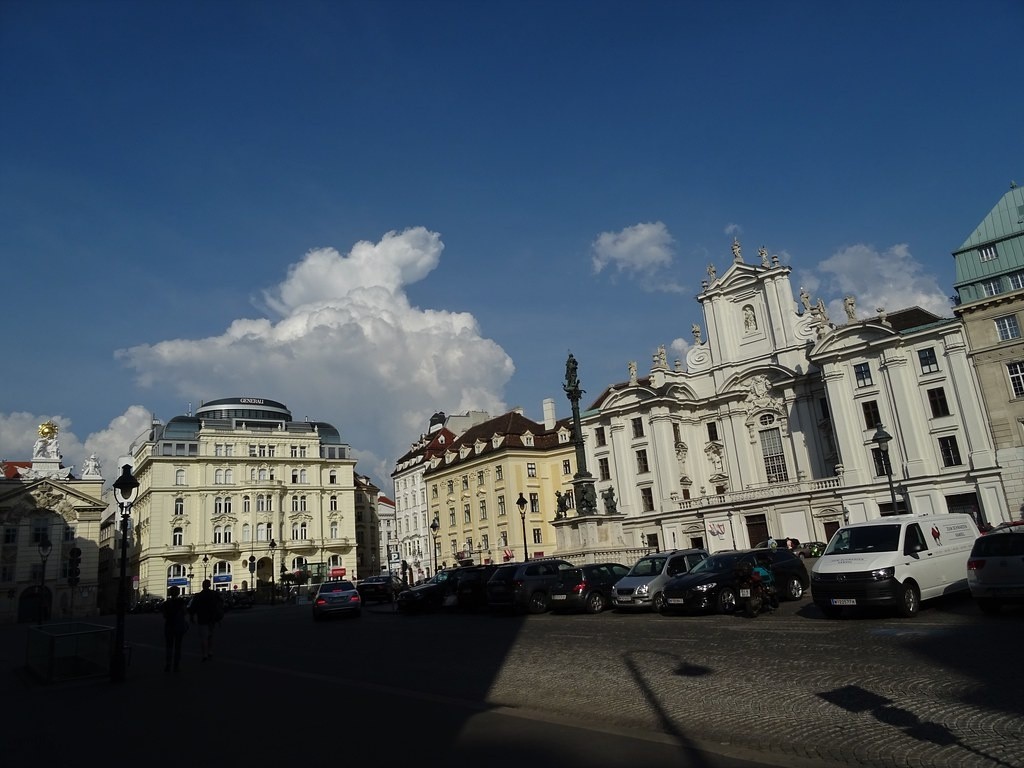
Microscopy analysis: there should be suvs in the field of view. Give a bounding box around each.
[660,547,810,616]
[487,560,576,614]
[396,565,490,614]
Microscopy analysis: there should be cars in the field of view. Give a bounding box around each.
[311,579,362,621]
[793,542,828,561]
[551,563,641,614]
[966,520,1024,615]
[356,575,408,605]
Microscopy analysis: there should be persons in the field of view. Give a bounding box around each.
[417,569,425,583]
[731,241,741,258]
[707,264,716,282]
[758,248,767,261]
[744,307,755,328]
[692,325,701,344]
[659,344,667,365]
[799,290,856,322]
[767,536,793,551]
[628,362,637,380]
[554,487,617,517]
[159,580,223,672]
[565,353,578,387]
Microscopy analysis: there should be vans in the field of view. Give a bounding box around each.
[610,549,711,613]
[754,539,802,554]
[811,512,983,618]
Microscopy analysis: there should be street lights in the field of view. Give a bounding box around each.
[202,554,209,580]
[430,519,440,583]
[268,539,277,603]
[37,533,53,629]
[106,464,141,684]
[640,533,645,547]
[872,423,899,515]
[726,510,737,550]
[515,493,529,562]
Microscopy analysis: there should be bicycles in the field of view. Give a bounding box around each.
[130,590,258,615]
